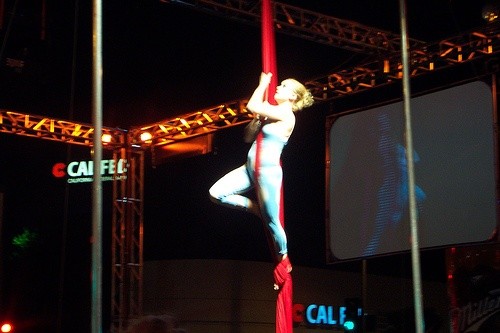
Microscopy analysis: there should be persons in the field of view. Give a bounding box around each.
[208,72,313,291]
